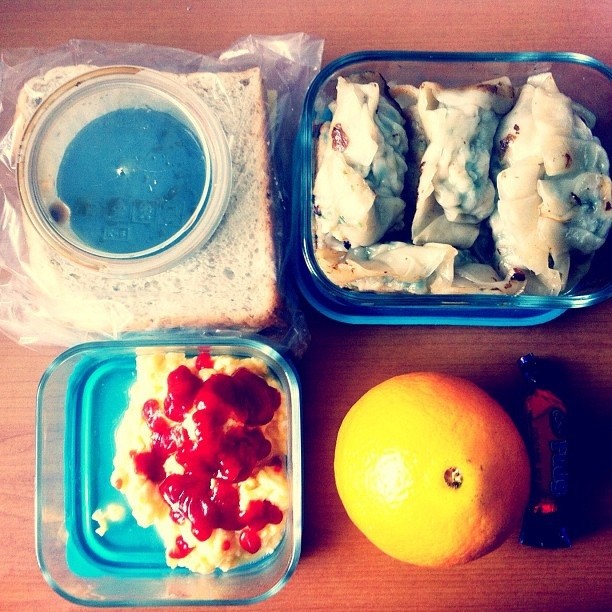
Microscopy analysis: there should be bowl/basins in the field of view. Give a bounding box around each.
[33,330,301,609]
[299,49,612,313]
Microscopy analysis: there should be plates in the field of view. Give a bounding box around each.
[298,273,581,328]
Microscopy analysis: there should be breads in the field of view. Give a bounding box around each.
[13,64,279,335]
